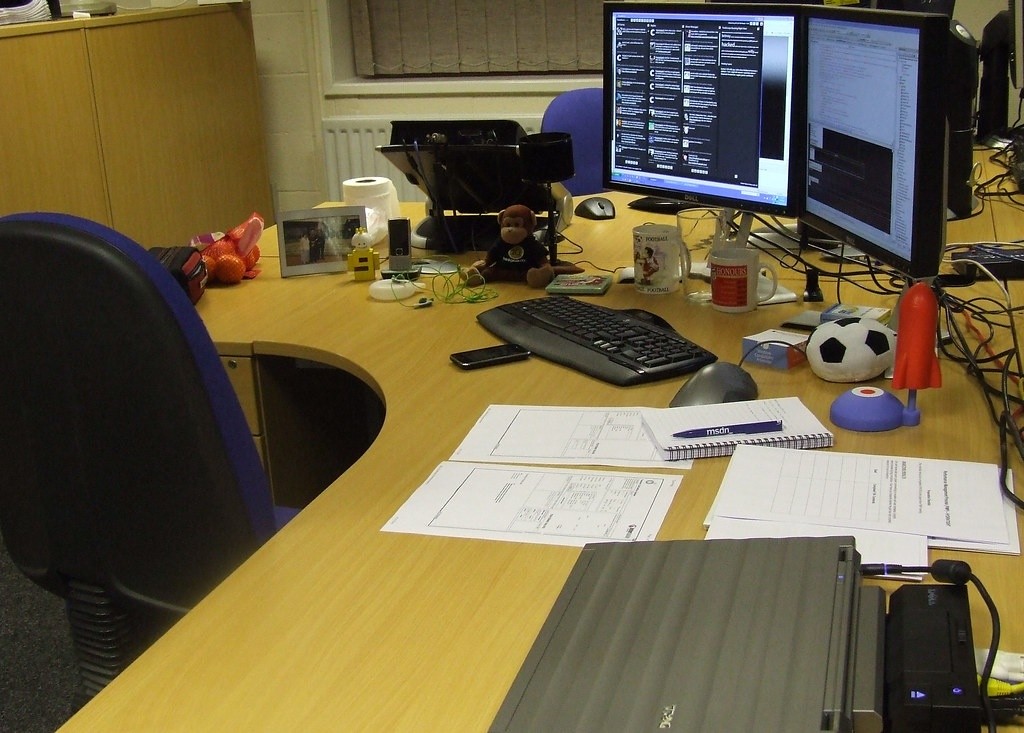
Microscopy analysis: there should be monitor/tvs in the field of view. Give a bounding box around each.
[602,0,801,219]
[799,4,949,277]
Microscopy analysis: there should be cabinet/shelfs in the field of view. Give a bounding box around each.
[0,0,275,252]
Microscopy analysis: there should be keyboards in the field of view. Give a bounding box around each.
[476,294,719,387]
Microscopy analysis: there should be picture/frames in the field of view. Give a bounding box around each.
[274,206,368,278]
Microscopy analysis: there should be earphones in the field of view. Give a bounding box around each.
[390,275,409,284]
[414,298,432,308]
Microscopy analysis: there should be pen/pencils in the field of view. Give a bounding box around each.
[670,420,783,438]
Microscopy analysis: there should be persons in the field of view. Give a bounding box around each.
[300,232,311,265]
[307,228,324,263]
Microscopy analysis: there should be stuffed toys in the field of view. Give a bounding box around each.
[458,204,585,289]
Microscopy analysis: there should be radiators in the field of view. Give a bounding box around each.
[322,115,544,203]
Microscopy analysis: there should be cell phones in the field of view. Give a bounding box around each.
[450,343,531,370]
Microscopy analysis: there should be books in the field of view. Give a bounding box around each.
[640,397,834,462]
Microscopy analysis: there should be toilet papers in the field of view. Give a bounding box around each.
[342,176,401,231]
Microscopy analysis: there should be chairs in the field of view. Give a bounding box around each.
[542,88,603,198]
[0,212,302,717]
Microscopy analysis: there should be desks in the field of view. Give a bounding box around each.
[53,141,1024,733]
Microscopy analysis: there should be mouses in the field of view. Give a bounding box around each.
[668,362,758,406]
[574,197,614,220]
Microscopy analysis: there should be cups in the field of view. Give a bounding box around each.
[632,225,691,294]
[709,247,777,313]
